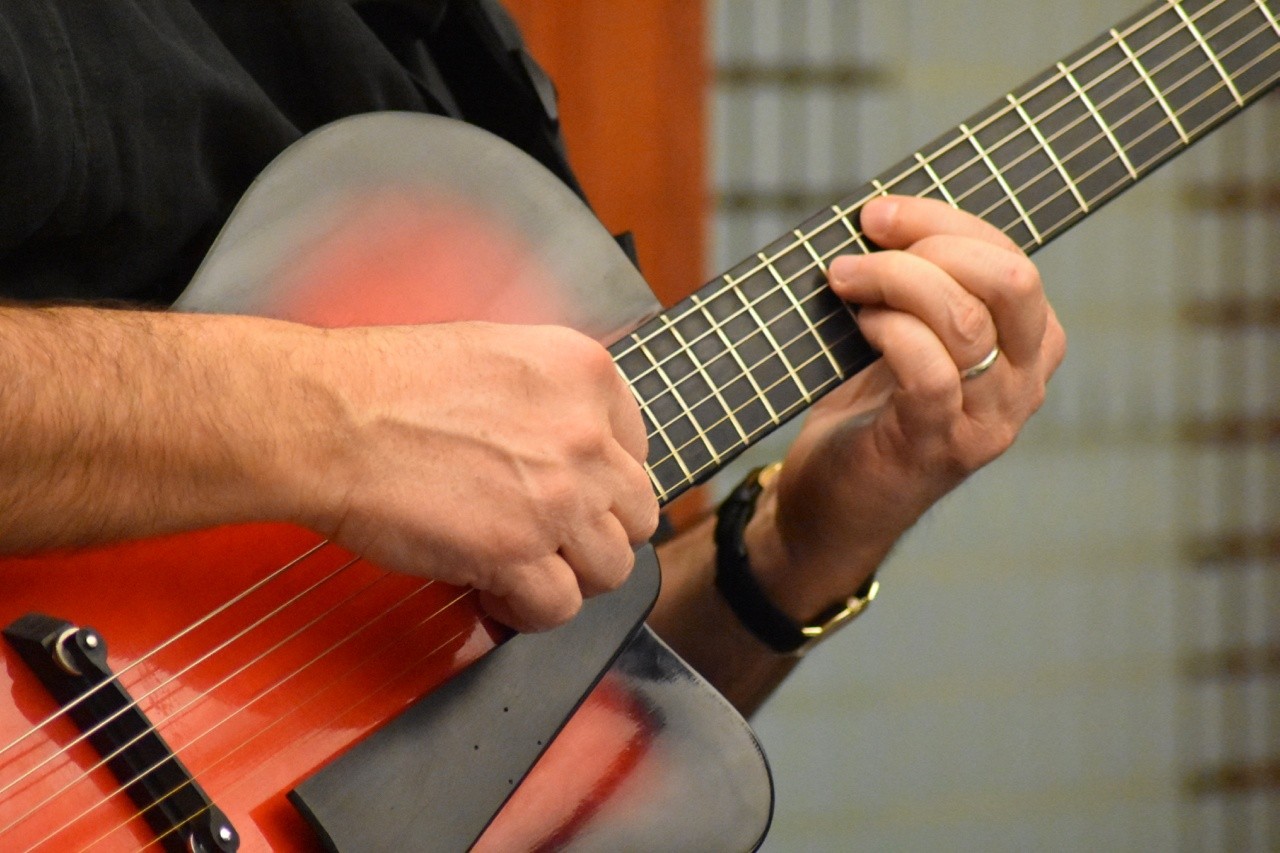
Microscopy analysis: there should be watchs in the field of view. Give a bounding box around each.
[707,452,885,663]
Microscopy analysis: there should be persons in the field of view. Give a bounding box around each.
[1,0,1071,722]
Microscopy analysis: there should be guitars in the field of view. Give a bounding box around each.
[1,1,1280,853]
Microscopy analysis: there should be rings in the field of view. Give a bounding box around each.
[960,344,1000,382]
